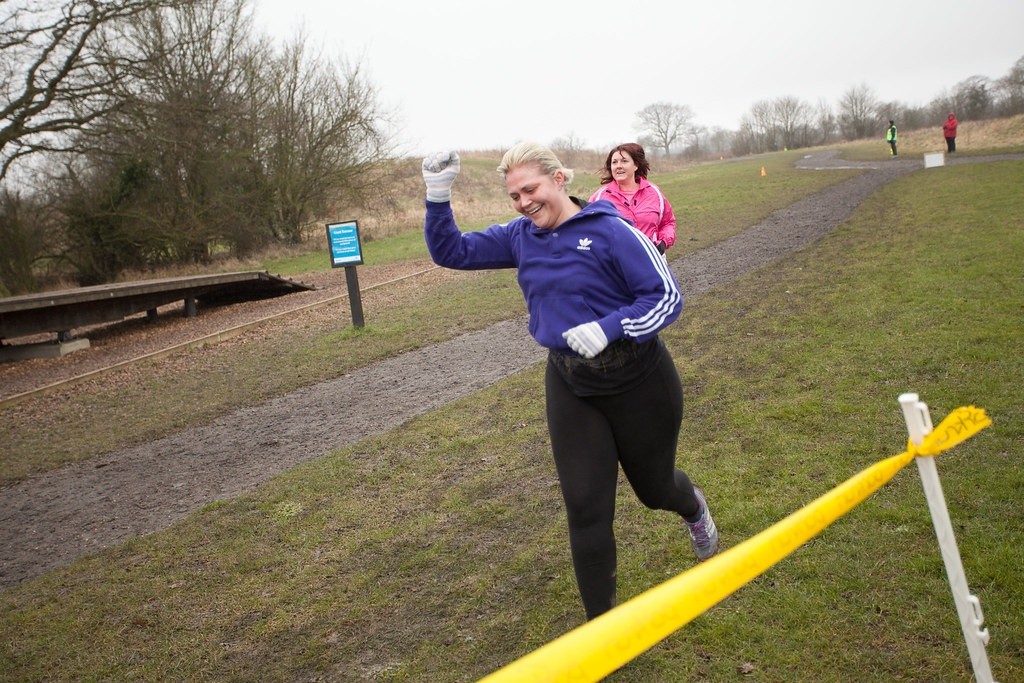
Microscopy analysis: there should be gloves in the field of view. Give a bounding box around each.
[561,321,608,360]
[422,148,461,203]
[652,240,665,256]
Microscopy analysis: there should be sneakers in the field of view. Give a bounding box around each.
[685,489,717,558]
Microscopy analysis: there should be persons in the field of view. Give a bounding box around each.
[942,111,958,154]
[421,141,717,624]
[588,143,677,266]
[887,120,898,158]
